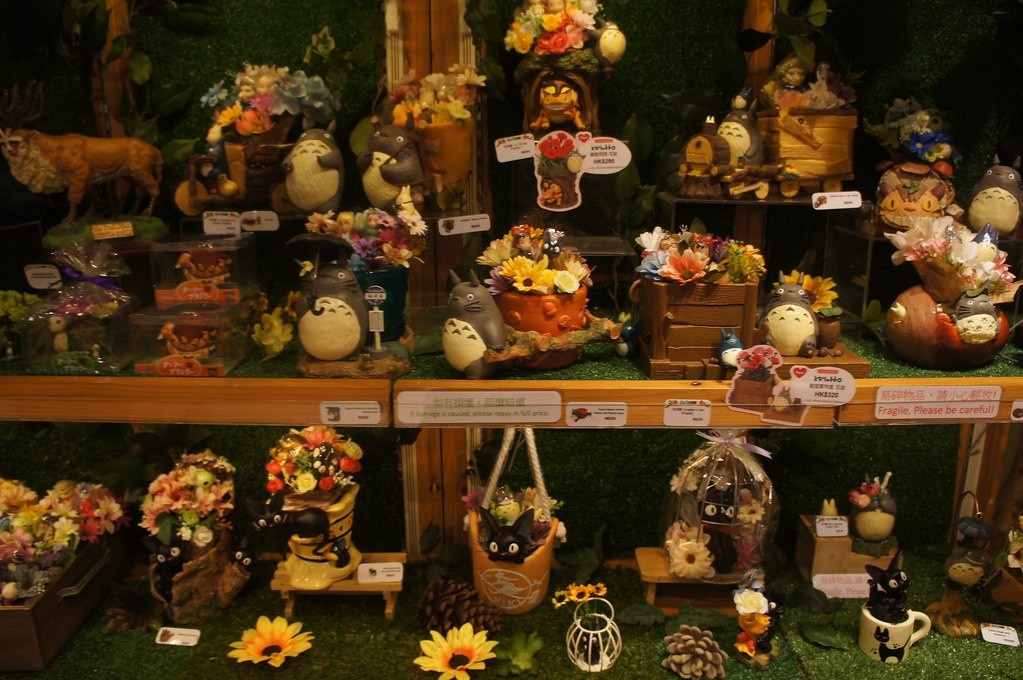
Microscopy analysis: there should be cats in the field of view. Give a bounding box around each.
[246,490,330,556]
[697,480,739,575]
[477,504,534,565]
[865,548,911,625]
[230,534,257,571]
[141,534,184,602]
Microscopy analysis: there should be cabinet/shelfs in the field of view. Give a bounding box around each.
[824,215,899,326]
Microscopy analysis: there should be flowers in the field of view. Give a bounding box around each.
[1,1,1022,679]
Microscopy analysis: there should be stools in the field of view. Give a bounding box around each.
[270,551,407,621]
[634,547,766,606]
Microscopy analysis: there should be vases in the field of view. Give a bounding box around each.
[567,599,625,672]
[344,264,409,342]
[856,507,897,541]
[814,305,846,350]
[628,277,759,379]
[0,533,125,671]
[225,114,342,205]
[420,117,475,187]
[274,482,364,590]
[886,285,1016,371]
[676,485,769,572]
[150,532,250,626]
[990,565,1022,604]
[855,217,880,239]
[494,269,590,370]
[473,517,560,614]
[758,109,858,179]
